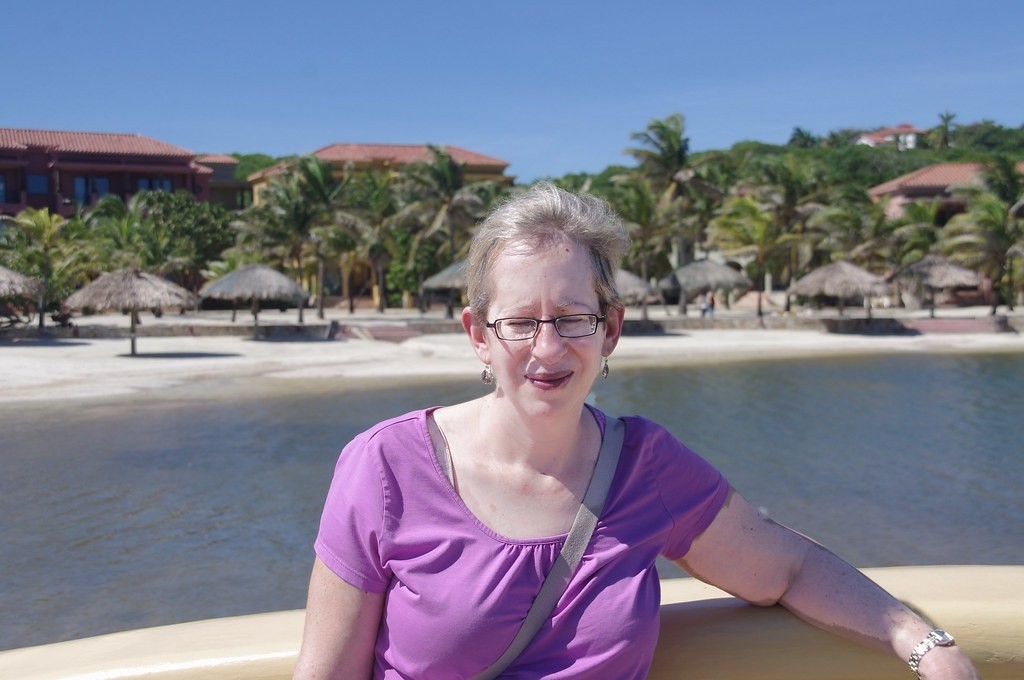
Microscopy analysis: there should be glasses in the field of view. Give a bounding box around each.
[483,314,607,341]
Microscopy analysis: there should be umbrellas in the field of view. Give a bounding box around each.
[0,265,45,297]
[658,258,751,319]
[198,265,309,321]
[617,268,657,297]
[65,267,202,353]
[885,254,981,318]
[790,259,893,319]
[424,261,469,288]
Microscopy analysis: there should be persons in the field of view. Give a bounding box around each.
[699,291,715,315]
[293,184,976,680]
[0,296,35,323]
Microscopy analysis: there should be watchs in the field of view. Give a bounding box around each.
[908,629,955,678]
